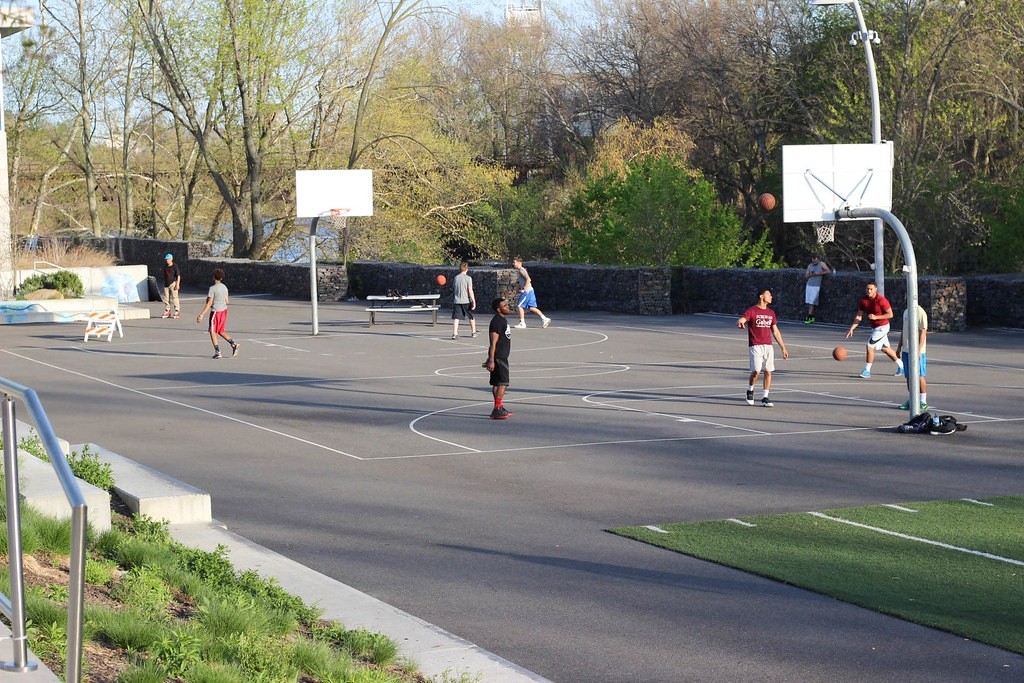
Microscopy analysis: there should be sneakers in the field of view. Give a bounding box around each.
[761,397,774,407]
[899,400,928,413]
[859,369,872,379]
[895,367,905,376]
[746,389,754,406]
[490,407,514,419]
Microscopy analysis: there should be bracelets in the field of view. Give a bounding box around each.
[199,314,203,318]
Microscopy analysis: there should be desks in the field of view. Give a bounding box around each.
[367,294,441,327]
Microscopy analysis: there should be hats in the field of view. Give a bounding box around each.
[165,254,173,259]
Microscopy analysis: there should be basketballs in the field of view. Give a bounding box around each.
[206,297,212,308]
[832,346,848,361]
[435,275,447,286]
[758,192,776,211]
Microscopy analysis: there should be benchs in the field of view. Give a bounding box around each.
[365,305,441,311]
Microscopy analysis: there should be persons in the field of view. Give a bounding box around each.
[805,257,831,324]
[845,281,904,378]
[897,291,929,413]
[512,256,551,329]
[482,298,514,420]
[162,253,180,319]
[197,269,239,358]
[450,262,480,340]
[737,289,788,407]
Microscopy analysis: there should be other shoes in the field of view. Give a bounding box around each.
[452,334,460,340]
[542,318,552,328]
[231,342,240,356]
[802,316,816,324]
[162,311,172,319]
[212,351,223,359]
[472,332,480,338]
[514,322,527,329]
[173,312,180,318]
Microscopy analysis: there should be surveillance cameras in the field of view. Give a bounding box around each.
[873,38,881,45]
[850,39,857,47]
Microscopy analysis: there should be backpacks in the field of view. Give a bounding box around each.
[898,412,931,432]
